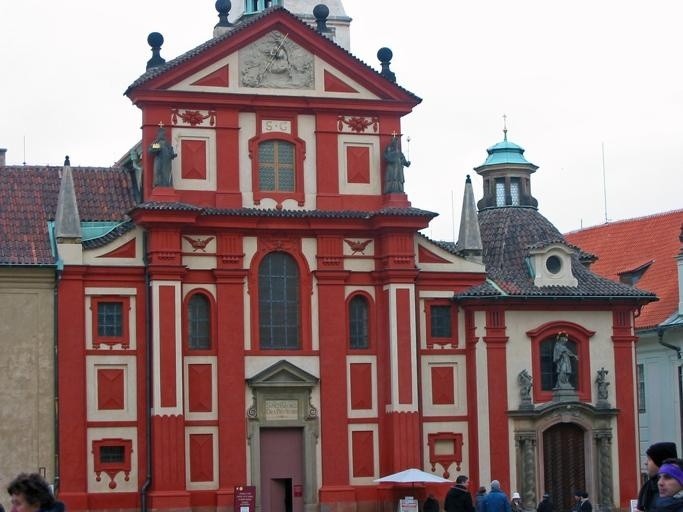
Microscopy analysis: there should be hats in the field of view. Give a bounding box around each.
[645,442,676,468]
[511,493,519,499]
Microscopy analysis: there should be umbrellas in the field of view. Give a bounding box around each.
[371,465,455,504]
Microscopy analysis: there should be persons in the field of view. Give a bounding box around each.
[381,134,412,196]
[146,125,178,190]
[636,441,683,511]
[6,472,67,510]
[647,458,682,511]
[422,473,594,511]
[551,328,582,401]
